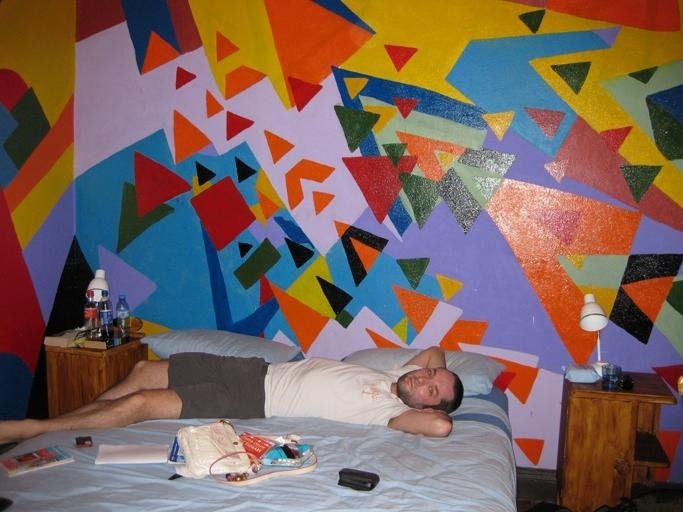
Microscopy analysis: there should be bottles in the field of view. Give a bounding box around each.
[83,289,102,338]
[116,294,130,343]
[96,290,114,347]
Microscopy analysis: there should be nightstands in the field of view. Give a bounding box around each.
[556,373,677,511]
[45,331,148,419]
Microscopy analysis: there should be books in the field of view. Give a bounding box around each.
[78,329,128,349]
[43,327,92,348]
[0,444,74,478]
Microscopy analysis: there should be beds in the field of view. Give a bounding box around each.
[1,386,516,512]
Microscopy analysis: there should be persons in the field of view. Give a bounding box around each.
[0,344,464,445]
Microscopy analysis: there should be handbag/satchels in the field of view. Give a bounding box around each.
[176,422,250,477]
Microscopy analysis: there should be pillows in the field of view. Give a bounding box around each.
[342,349,506,394]
[140,330,301,363]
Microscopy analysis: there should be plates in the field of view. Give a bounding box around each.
[126,316,142,333]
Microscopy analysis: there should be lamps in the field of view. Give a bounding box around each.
[85,269,110,302]
[580,293,608,377]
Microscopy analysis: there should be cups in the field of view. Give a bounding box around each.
[601,364,622,390]
